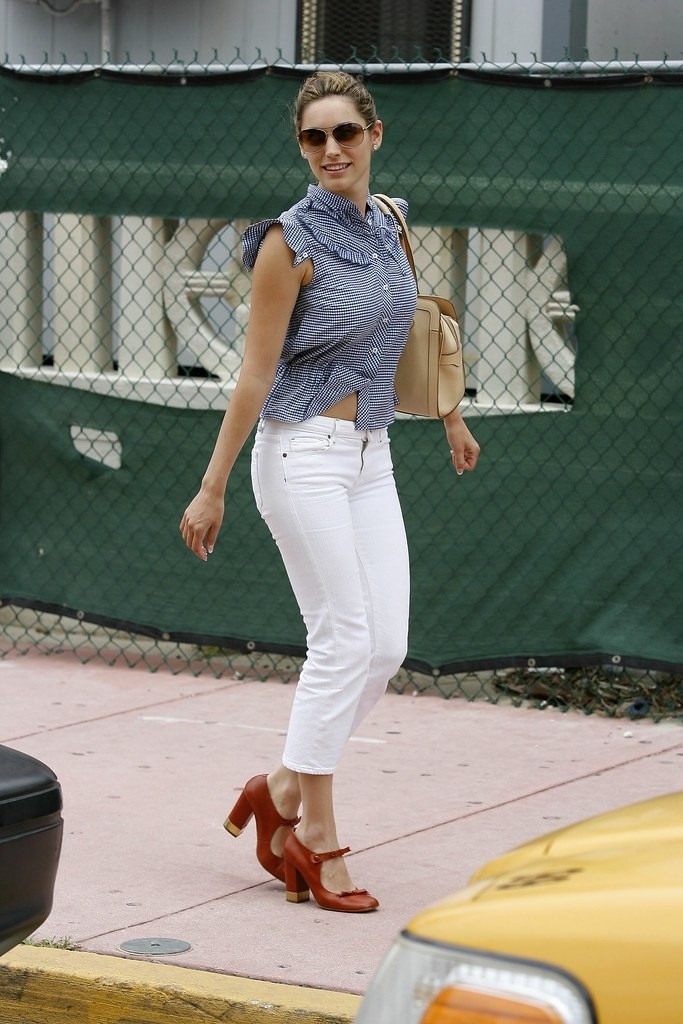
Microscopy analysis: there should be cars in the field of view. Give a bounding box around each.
[356,790,683,1022]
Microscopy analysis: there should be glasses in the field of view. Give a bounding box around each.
[297,122,374,154]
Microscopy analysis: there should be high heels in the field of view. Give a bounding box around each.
[223,772,302,884]
[283,829,380,914]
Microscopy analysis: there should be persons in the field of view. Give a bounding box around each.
[177,70,482,913]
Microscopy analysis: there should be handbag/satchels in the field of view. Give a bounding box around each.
[394,295,467,418]
